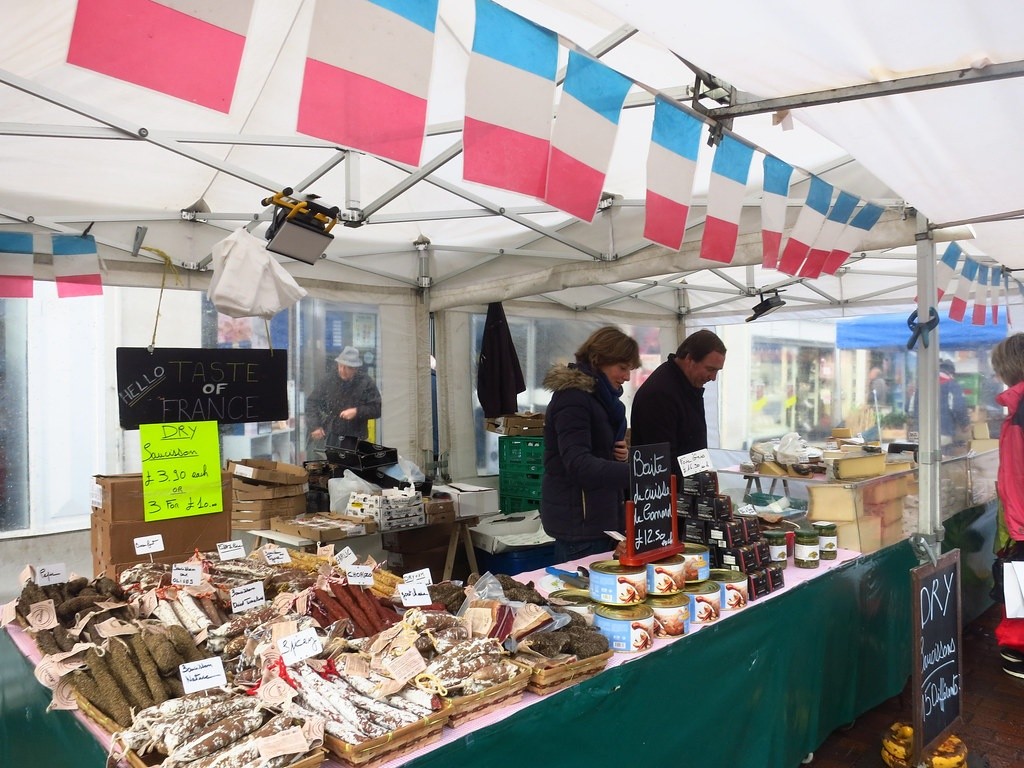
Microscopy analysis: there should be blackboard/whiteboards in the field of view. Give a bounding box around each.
[619,440,686,568]
[908,547,966,765]
[114,346,292,433]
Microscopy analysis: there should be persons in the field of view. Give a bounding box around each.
[304,345,383,441]
[914,359,972,448]
[538,324,634,562]
[868,365,888,406]
[631,326,727,492]
[990,330,1024,680]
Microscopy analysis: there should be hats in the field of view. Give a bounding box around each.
[333,346,363,368]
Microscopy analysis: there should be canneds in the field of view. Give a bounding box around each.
[762,520,837,570]
[548,542,749,653]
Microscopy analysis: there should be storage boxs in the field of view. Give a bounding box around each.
[91,409,557,586]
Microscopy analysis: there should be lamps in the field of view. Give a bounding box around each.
[745,296,787,322]
[265,207,335,266]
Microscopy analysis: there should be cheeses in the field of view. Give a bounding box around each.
[739,427,919,552]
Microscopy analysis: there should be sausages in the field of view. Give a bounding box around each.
[14,542,608,768]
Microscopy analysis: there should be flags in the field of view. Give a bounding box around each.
[972,262,989,326]
[538,49,635,226]
[460,1,561,201]
[913,240,964,305]
[1003,273,1014,326]
[65,0,255,113]
[822,200,886,275]
[991,265,1002,324]
[50,223,105,299]
[295,3,440,168]
[946,254,979,324]
[642,92,707,253]
[761,153,794,270]
[0,230,33,300]
[780,175,834,278]
[699,132,758,266]
[800,189,863,280]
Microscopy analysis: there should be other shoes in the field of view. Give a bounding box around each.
[999,647,1024,679]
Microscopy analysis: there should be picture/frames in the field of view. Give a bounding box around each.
[910,548,965,767]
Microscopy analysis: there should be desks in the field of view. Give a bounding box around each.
[0,448,1001,768]
[253,516,482,585]
